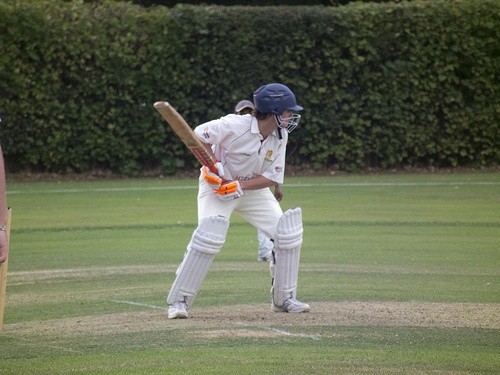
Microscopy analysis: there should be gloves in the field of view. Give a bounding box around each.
[200,161,224,190]
[217,179,243,201]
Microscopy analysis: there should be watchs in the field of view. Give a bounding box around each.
[0,223,7,231]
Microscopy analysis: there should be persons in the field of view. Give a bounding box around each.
[0,147,13,336]
[235,99,284,261]
[166,84,311,320]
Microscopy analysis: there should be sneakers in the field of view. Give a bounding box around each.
[167,301,188,318]
[271,298,310,313]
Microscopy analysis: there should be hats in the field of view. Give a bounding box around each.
[235,99,255,112]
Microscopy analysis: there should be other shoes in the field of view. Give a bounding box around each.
[258,251,275,261]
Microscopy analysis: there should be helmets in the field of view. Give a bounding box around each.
[253,83,303,115]
